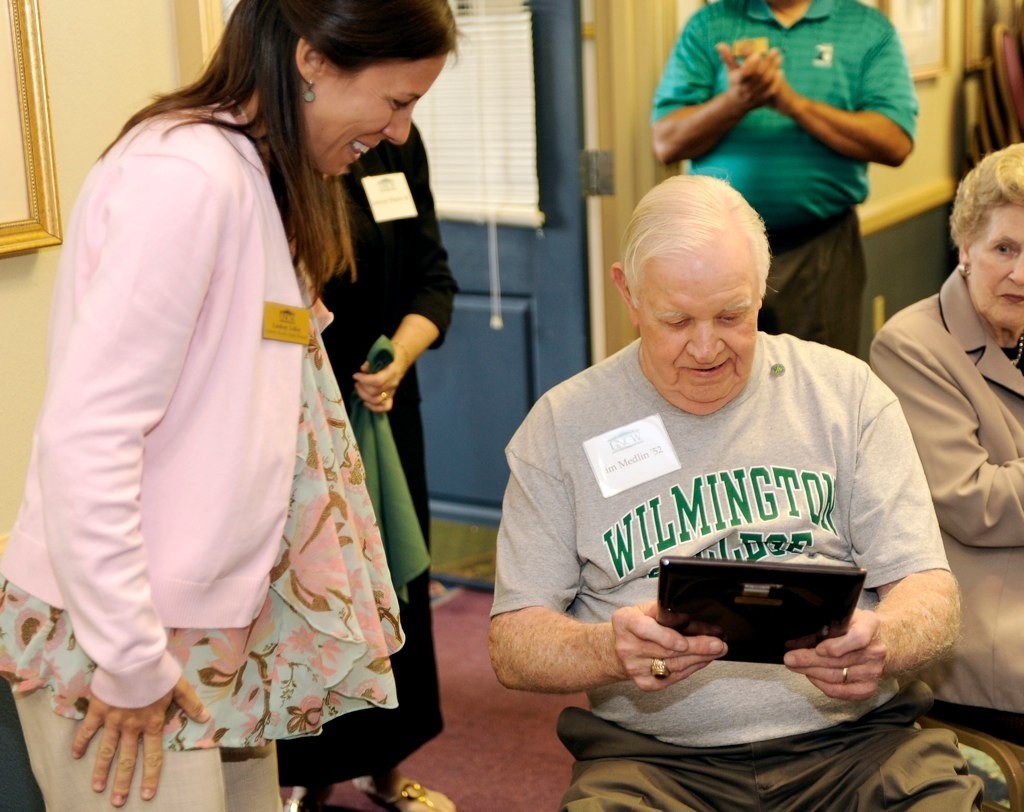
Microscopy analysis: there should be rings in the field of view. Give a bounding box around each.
[651,657,670,680]
[382,392,387,400]
[842,668,848,685]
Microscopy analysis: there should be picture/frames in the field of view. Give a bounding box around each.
[0,0,65,261]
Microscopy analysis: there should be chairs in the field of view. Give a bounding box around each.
[964,19,1024,163]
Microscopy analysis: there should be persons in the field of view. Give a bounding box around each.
[651,0,917,359]
[487,174,985,812]
[279,116,458,812]
[0,0,459,812]
[869,143,1024,713]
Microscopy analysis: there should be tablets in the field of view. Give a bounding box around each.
[655,557,868,664]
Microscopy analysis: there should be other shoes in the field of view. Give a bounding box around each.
[283,783,332,812]
[353,774,457,812]
